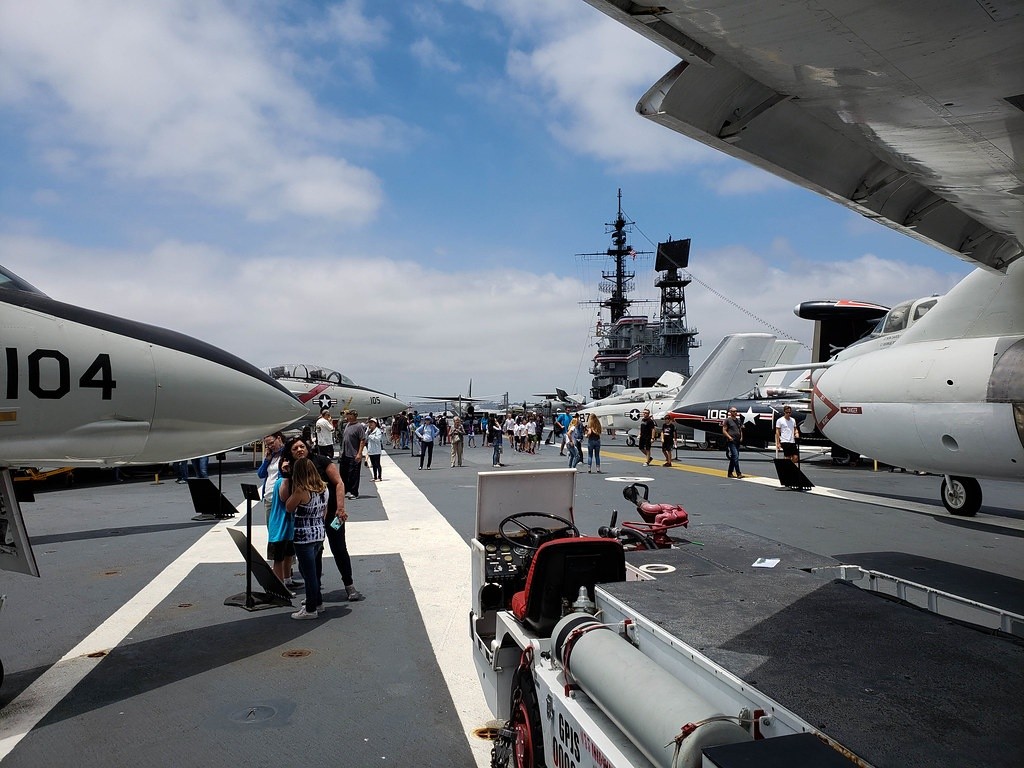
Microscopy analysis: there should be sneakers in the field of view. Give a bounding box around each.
[291,603,326,619]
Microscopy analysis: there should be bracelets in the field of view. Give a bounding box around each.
[651,438,654,439]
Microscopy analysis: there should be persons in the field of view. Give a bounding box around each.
[723,407,745,478]
[316,409,334,459]
[637,409,656,466]
[660,415,676,467]
[776,406,800,462]
[553,408,602,473]
[385,411,464,470]
[465,403,545,468]
[173,456,209,483]
[338,410,382,498]
[607,429,612,435]
[257,432,361,620]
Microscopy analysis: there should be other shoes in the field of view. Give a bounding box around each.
[344,584,360,600]
[560,452,566,456]
[643,456,654,467]
[369,479,382,482]
[663,463,672,467]
[178,480,188,485]
[728,474,745,479]
[510,444,535,455]
[468,445,477,448]
[286,580,306,597]
[418,467,431,470]
[578,461,583,465]
[493,462,504,468]
[344,491,359,500]
[451,464,461,468]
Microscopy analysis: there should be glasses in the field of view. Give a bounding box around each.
[784,410,792,414]
[665,417,671,420]
[265,436,279,447]
[286,434,303,443]
[730,410,738,413]
[642,411,650,414]
[566,409,572,412]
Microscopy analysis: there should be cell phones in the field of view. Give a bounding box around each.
[330,516,345,530]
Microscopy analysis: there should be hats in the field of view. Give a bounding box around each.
[347,410,358,417]
[368,418,377,423]
[425,416,432,422]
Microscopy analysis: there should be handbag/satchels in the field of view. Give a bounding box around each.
[451,430,460,443]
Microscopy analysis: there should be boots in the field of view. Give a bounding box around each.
[587,464,602,473]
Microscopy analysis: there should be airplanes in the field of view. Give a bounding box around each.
[527,294,942,466]
[582,0,1023,517]
[260,364,411,435]
[0,265,309,578]
